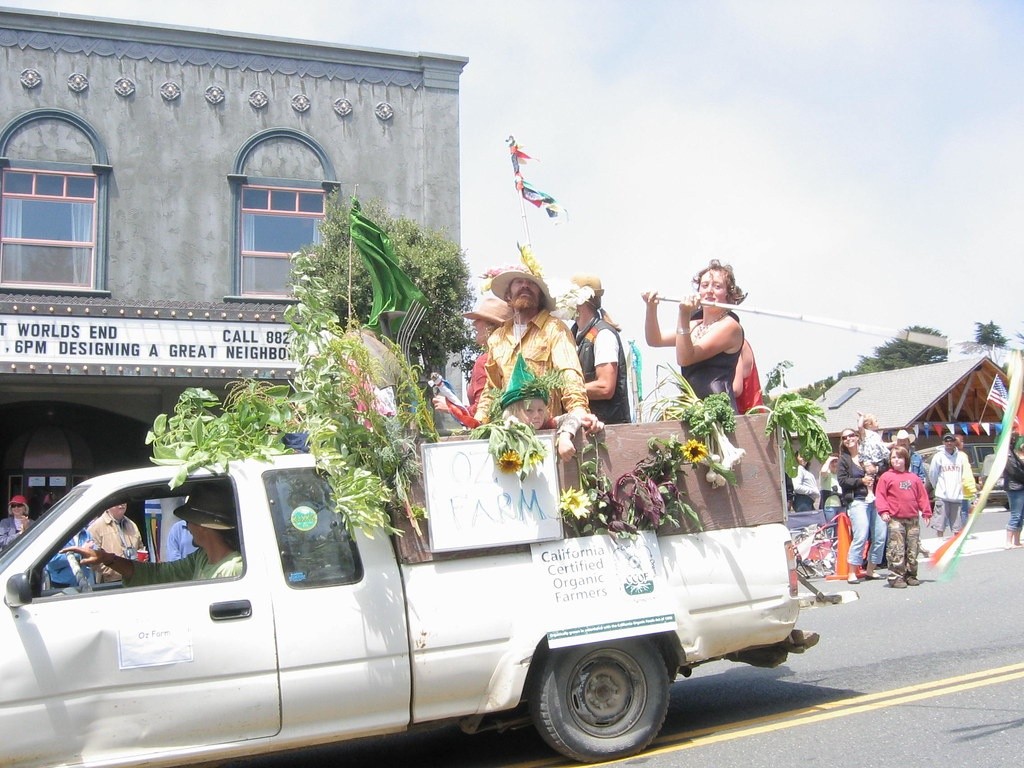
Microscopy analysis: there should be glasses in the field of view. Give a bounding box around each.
[11,504,24,508]
[943,438,954,443]
[841,433,856,441]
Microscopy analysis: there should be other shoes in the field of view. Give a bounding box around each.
[907,576,920,586]
[847,576,860,584]
[1005,542,1024,549]
[889,579,908,587]
[865,495,877,504]
[865,571,886,580]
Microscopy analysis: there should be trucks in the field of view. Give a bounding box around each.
[0,450,860,768]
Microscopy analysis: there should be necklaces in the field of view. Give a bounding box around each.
[692,311,727,340]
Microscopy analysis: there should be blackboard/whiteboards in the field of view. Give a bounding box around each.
[421,435,564,553]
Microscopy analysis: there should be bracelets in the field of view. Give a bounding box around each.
[676,326,691,334]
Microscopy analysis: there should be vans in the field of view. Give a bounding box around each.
[913,443,1011,511]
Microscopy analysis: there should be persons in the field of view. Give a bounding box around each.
[44,527,95,590]
[165,521,200,563]
[431,263,766,461]
[1003,433,1024,550]
[340,329,399,434]
[0,494,35,553]
[87,502,150,583]
[784,413,976,589]
[59,484,243,588]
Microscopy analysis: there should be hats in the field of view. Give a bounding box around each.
[490,266,557,311]
[8,495,27,506]
[890,429,916,445]
[499,353,549,409]
[173,481,238,530]
[462,294,507,326]
[572,278,604,297]
[943,433,955,440]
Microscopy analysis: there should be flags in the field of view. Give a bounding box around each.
[989,376,1009,413]
